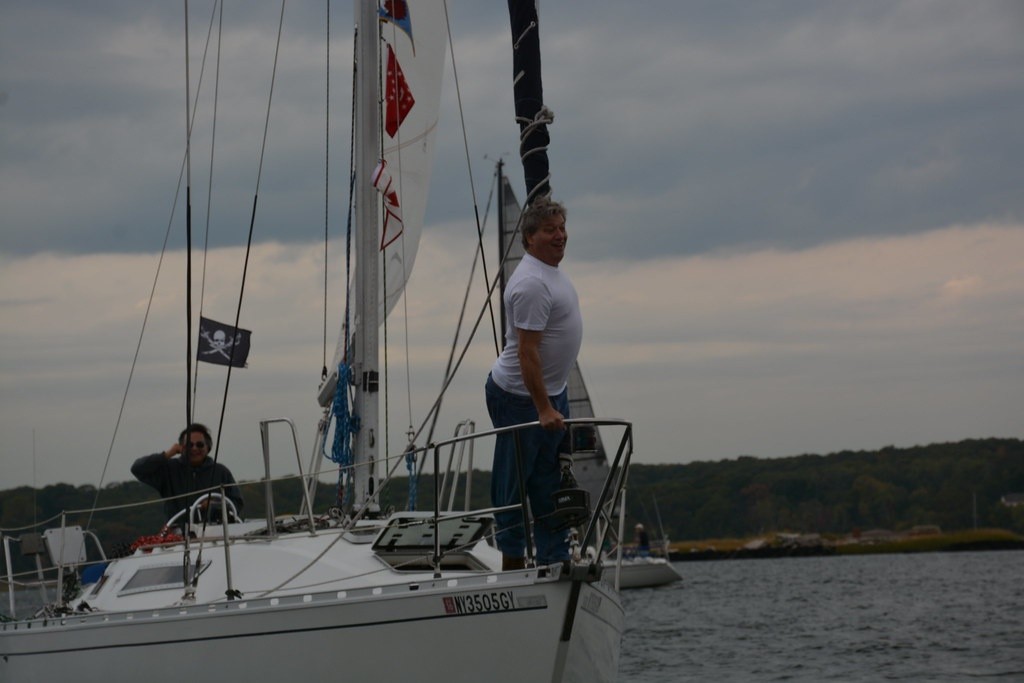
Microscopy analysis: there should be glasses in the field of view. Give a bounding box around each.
[190,441,208,448]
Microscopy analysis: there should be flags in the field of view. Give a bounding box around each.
[198,316,252,371]
[369,0,415,250]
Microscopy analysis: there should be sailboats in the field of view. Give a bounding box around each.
[402,158,682,589]
[0,11,635,682]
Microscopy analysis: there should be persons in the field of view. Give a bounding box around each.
[485,196,585,570]
[634,522,650,558]
[130,423,244,526]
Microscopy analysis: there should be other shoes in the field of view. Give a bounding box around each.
[536,562,596,578]
[502,554,526,571]
